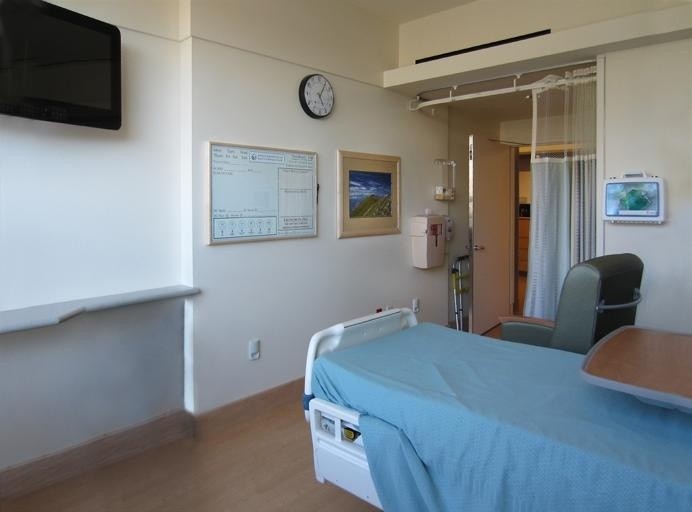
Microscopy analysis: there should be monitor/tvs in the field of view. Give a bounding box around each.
[0,0,122,131]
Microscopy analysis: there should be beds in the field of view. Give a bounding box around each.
[301,305,692,512]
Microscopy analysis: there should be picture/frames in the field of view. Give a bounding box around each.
[335,149,403,240]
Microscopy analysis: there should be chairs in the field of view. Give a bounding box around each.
[496,252,647,357]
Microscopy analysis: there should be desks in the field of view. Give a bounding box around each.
[578,322,691,412]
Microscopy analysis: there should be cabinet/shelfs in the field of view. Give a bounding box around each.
[516,217,530,272]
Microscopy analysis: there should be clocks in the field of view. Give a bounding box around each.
[297,71,337,120]
